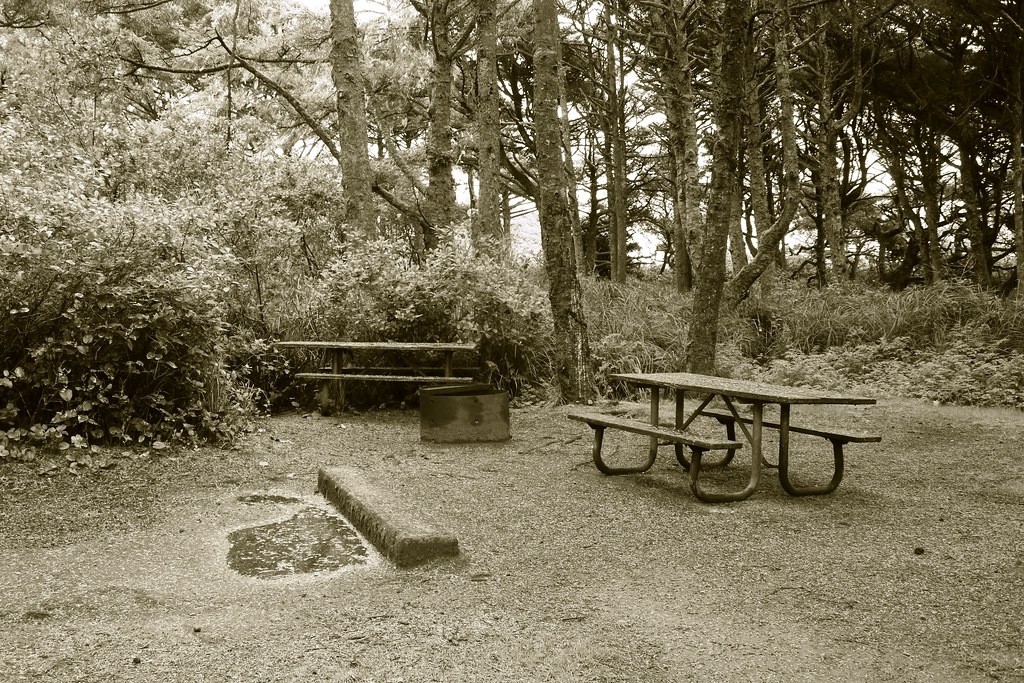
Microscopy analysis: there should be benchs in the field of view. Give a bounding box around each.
[294,373,473,418]
[566,413,743,504]
[319,365,481,406]
[696,408,881,494]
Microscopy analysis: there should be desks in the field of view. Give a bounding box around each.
[608,374,878,501]
[278,341,479,410]
